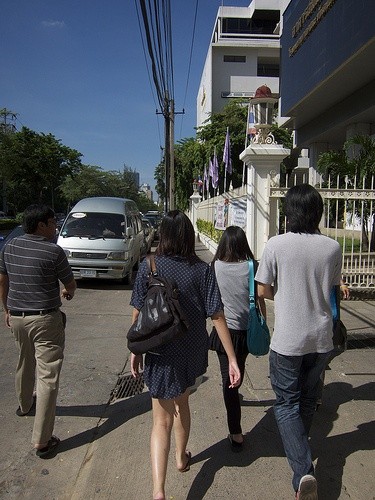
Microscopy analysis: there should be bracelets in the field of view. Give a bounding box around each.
[340,285,347,289]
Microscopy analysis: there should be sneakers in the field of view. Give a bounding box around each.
[295,474,319,500]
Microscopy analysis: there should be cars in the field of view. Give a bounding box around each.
[139,210,162,256]
[0,225,25,250]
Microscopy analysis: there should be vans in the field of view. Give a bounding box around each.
[52,197,147,284]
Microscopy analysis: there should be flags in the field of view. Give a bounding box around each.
[199,108,254,190]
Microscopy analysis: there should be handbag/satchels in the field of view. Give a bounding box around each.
[127,255,191,355]
[332,317,347,353]
[246,259,270,356]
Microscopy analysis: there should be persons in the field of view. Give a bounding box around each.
[252,184,351,500]
[129,210,241,500]
[0,205,77,455]
[209,225,269,449]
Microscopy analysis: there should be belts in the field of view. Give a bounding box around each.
[7,307,59,316]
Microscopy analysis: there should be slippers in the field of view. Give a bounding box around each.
[177,450,191,471]
[227,432,245,453]
[36,435,61,456]
[16,390,36,416]
